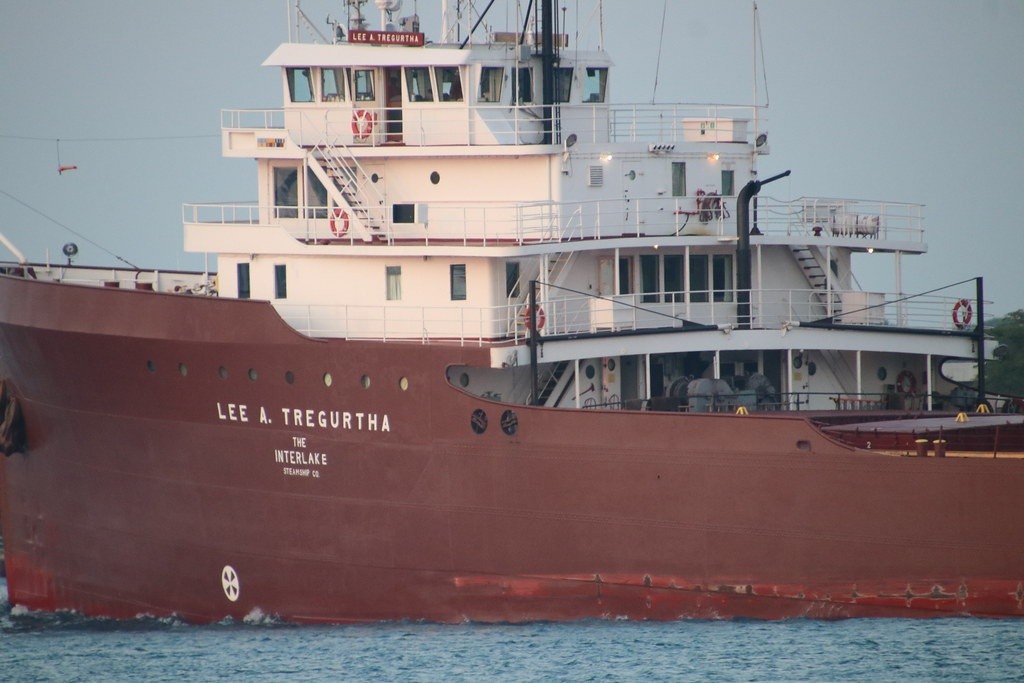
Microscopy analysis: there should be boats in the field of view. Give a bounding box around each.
[0,0,1023,625]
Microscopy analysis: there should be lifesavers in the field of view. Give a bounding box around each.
[952,298,973,329]
[351,109,373,140]
[524,304,545,333]
[896,369,918,400]
[328,208,350,238]
